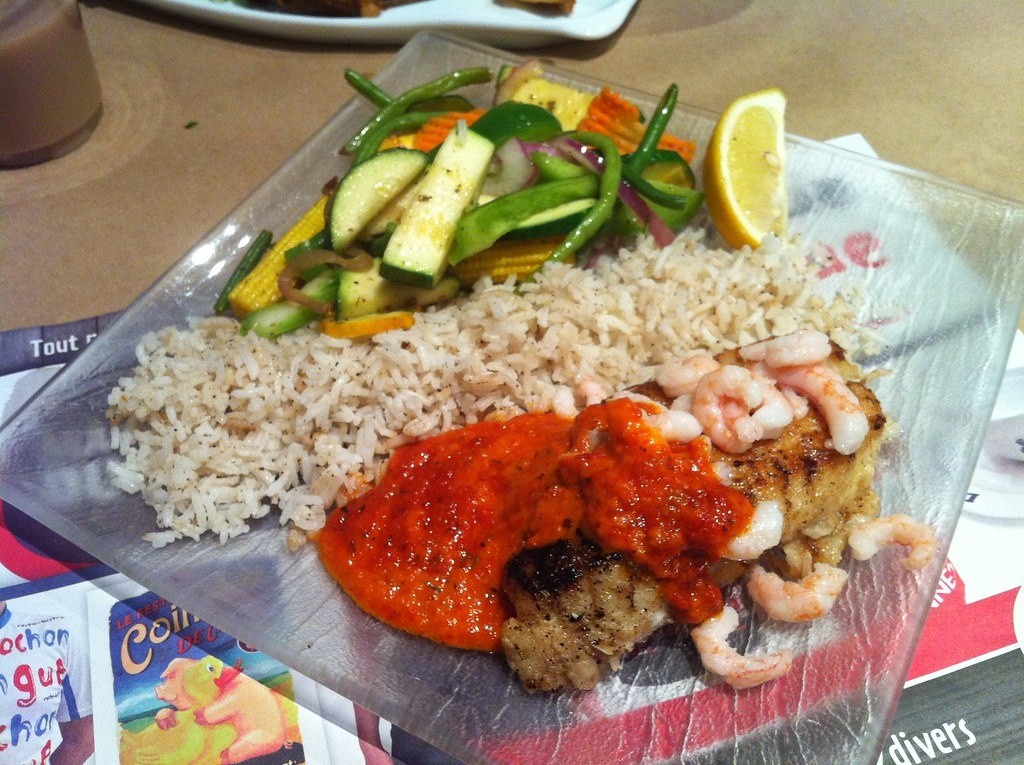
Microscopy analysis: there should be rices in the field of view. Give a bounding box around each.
[110,226,888,549]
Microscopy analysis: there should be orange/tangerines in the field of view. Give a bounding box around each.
[706,88,786,249]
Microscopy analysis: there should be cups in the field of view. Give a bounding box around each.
[0,1,104,173]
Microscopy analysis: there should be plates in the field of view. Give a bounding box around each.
[139,0,640,49]
[0,25,1024,764]
